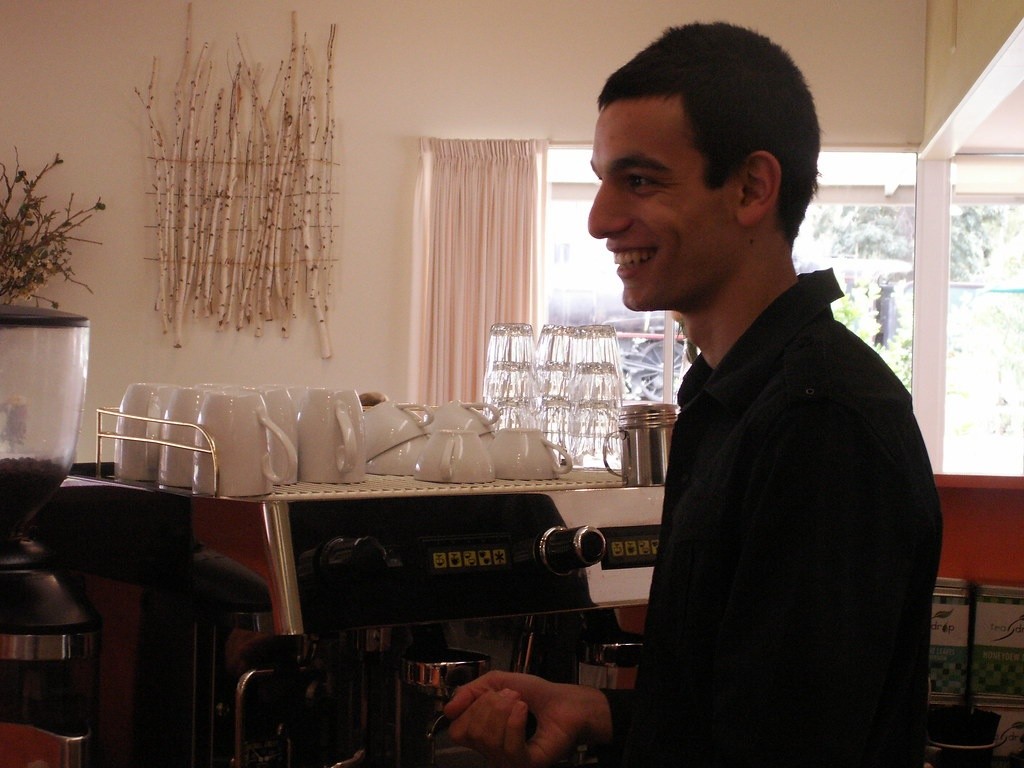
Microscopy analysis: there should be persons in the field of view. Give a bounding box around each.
[441,22,943,768]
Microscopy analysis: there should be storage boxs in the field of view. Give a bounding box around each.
[970,577,1024,697]
[929,577,974,696]
[972,695,1024,768]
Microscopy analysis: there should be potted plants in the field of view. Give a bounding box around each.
[0,142,105,475]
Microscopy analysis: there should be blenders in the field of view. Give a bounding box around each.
[0,303,100,738]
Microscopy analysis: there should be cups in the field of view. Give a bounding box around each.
[604,403,677,489]
[115,323,677,497]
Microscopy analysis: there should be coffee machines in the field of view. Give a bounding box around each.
[105,465,663,768]
